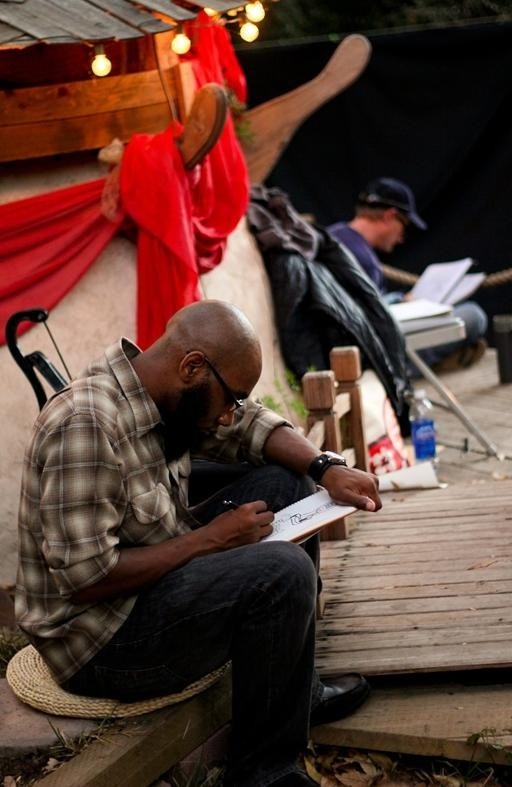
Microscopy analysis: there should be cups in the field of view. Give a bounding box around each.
[489,313,511,383]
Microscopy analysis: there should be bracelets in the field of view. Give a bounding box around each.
[309,448,348,484]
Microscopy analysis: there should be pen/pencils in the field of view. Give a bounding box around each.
[222,500,239,509]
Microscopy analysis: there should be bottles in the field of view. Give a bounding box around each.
[408,387,440,476]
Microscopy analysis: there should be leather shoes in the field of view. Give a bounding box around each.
[310,673,372,724]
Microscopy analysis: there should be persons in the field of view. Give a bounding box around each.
[317,177,491,376]
[17,298,382,785]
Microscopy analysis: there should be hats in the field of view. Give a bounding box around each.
[360,176,427,231]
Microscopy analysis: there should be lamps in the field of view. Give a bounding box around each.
[91,0,266,78]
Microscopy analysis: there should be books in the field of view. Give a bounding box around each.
[388,257,487,332]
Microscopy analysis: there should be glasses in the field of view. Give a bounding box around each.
[200,357,244,413]
